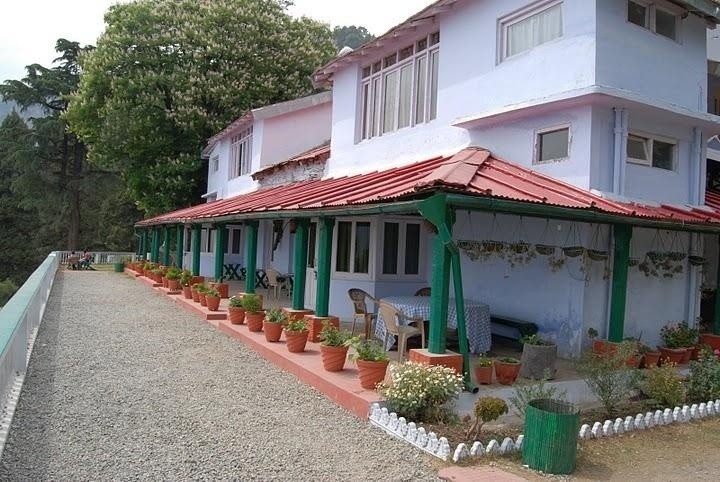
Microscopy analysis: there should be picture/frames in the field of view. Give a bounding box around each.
[373,293,492,353]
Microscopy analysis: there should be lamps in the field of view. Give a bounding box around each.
[376,302,426,361]
[67,253,97,271]
[347,289,380,339]
[413,286,432,336]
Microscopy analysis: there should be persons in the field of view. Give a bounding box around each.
[69,250,78,268]
[79,250,89,265]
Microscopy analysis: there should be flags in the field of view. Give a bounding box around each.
[474,366,494,386]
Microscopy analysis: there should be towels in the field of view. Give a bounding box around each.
[521,399,581,475]
[114,263,124,272]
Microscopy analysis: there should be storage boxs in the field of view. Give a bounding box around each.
[485,313,539,352]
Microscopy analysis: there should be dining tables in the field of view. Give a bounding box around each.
[515,330,559,380]
[494,353,528,385]
[583,323,719,369]
[121,258,385,392]
[456,237,712,283]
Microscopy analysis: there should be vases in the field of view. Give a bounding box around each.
[473,350,493,367]
